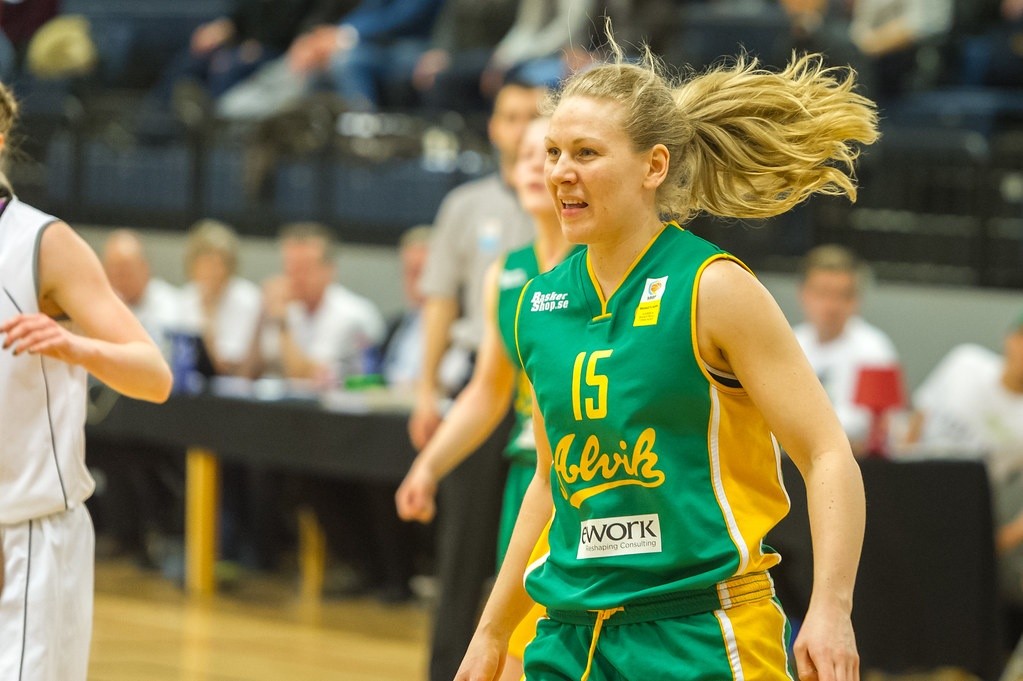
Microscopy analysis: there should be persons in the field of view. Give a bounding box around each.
[96,221,474,603]
[451,62,866,681]
[389,59,586,681]
[1,84,174,681]
[792,242,903,458]
[843,1,960,100]
[895,321,1023,595]
[132,0,602,113]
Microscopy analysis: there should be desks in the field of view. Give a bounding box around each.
[80,365,1023,609]
